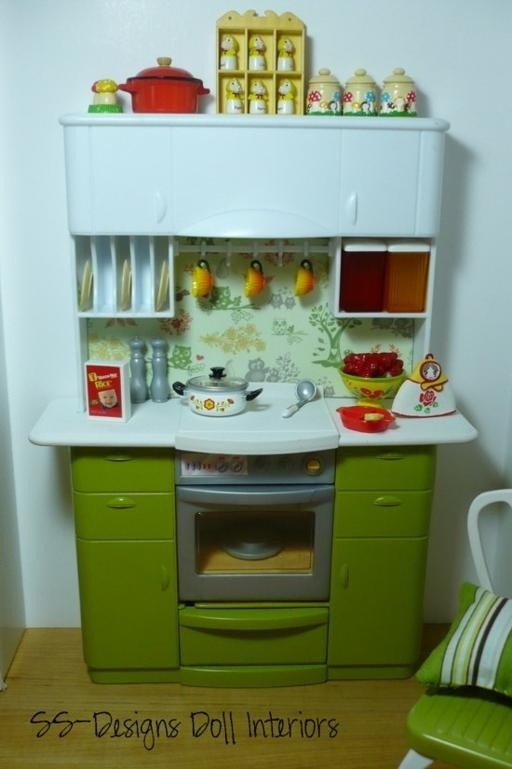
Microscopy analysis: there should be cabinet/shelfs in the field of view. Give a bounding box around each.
[26,113,480,690]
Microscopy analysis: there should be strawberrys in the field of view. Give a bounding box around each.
[344,352,404,377]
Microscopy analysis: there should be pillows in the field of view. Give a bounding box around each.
[414,580,511,698]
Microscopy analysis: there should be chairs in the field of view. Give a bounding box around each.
[397,487,512,769]
